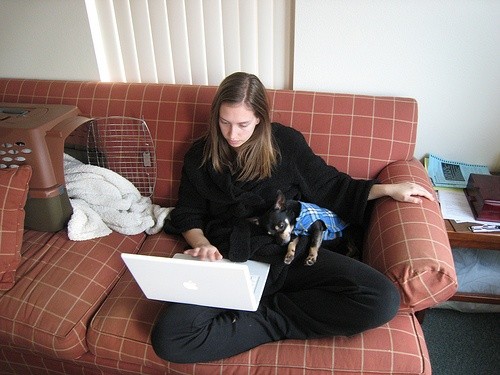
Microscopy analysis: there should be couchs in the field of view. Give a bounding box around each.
[0,77,459,375]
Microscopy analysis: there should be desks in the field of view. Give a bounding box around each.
[414,172,500,325]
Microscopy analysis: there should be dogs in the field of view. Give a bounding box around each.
[247,190,358,267]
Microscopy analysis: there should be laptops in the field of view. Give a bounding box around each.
[120,252,271,311]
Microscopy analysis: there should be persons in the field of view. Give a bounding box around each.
[151,72,435,363]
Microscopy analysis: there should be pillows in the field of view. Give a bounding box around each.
[0,165,32,290]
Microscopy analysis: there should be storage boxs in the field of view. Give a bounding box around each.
[464,173,500,223]
